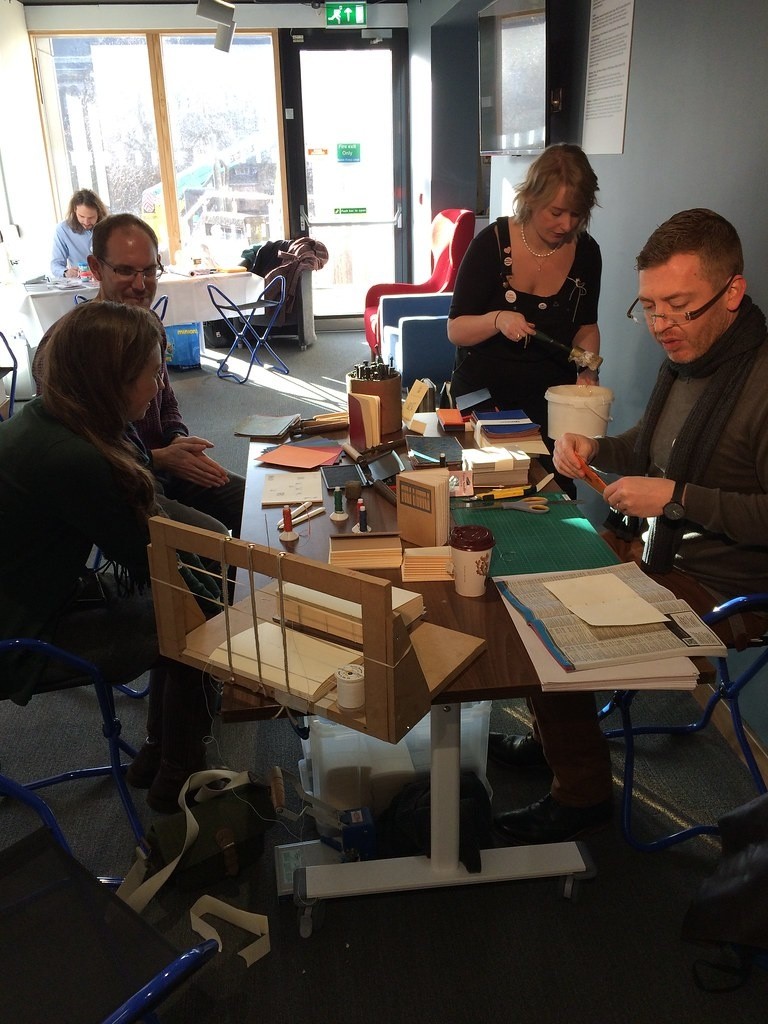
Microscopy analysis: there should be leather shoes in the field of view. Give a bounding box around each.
[487,731,558,772]
[489,790,617,846]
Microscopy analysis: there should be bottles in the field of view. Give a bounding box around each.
[78,262,90,282]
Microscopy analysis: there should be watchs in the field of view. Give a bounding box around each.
[663,480,686,522]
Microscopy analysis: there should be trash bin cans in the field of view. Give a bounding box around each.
[203,318,235,349]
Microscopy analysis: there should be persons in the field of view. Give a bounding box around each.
[50,189,107,279]
[0,302,234,812]
[446,142,602,508]
[31,214,245,612]
[486,208,768,845]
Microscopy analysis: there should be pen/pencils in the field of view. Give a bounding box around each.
[277,502,312,525]
[279,507,326,529]
[439,452,448,468]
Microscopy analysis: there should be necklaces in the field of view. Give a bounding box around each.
[530,254,544,271]
[521,219,565,257]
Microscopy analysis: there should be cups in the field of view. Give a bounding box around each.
[450,524,496,597]
[345,370,402,435]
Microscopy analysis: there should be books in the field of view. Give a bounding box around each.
[209,584,424,701]
[396,467,448,546]
[328,531,403,570]
[493,560,729,693]
[261,471,324,504]
[408,408,550,487]
[234,412,302,438]
[418,378,437,410]
[401,546,454,581]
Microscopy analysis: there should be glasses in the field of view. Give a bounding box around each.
[626,274,739,326]
[94,255,158,279]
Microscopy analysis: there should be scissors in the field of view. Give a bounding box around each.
[469,497,550,514]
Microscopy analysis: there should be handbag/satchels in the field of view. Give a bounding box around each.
[161,321,203,373]
[202,318,234,348]
[139,779,279,898]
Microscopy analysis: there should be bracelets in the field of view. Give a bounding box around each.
[494,310,502,331]
[63,270,67,278]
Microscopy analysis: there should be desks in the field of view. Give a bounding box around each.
[222,413,716,938]
[22,264,265,338]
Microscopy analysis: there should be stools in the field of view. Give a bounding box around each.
[207,275,290,384]
[0,332,18,422]
[75,294,168,321]
[599,593,768,853]
[1,774,219,1024]
[0,639,152,915]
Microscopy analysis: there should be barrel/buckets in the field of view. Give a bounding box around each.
[544,384,614,441]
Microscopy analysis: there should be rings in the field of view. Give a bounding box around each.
[517,334,520,339]
[616,503,623,512]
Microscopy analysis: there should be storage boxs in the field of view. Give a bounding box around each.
[298,699,493,836]
[0,395,10,420]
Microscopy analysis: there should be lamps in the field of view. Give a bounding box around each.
[195,0,237,52]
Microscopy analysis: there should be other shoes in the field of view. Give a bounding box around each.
[125,734,235,815]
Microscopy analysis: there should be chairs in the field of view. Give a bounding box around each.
[364,209,476,361]
[379,293,457,394]
